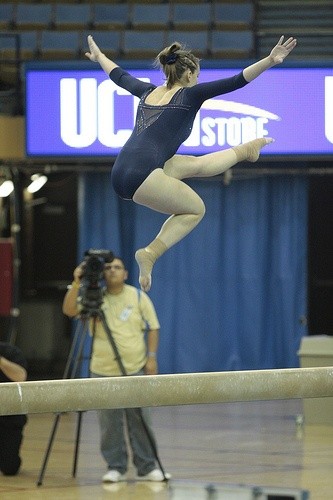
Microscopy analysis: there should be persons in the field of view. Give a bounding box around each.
[85,31,298,291]
[0,340,30,475]
[61,258,171,484]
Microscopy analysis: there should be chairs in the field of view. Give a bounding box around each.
[0,0,257,87]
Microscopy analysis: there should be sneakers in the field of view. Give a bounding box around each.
[136,469,171,481]
[101,469,127,482]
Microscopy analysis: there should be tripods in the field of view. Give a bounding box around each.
[37,278,169,487]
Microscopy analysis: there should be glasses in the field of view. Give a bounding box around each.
[104,265,125,270]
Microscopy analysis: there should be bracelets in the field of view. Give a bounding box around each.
[66,282,84,290]
[148,352,157,357]
